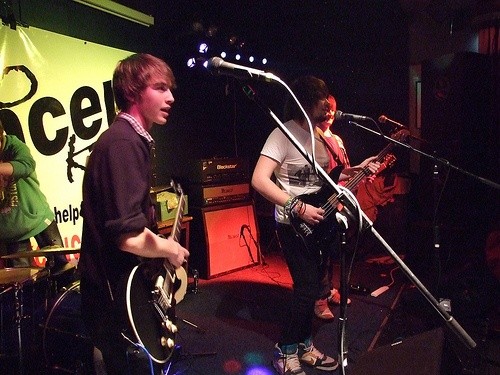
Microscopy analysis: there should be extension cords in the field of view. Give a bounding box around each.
[370,285,389,297]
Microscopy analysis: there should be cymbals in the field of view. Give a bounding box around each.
[1,244,81,259]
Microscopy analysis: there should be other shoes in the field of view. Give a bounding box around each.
[313,298,334,320]
[328,288,352,305]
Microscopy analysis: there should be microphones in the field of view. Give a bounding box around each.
[378,115,406,129]
[210,56,265,76]
[334,110,368,120]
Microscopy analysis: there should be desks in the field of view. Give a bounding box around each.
[156,216,193,275]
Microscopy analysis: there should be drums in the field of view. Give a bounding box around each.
[42,281,82,375]
[0,266,59,375]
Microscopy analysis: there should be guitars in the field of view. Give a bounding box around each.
[120,184,189,364]
[287,129,410,268]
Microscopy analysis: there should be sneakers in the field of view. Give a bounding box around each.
[298,343,339,371]
[272,343,305,375]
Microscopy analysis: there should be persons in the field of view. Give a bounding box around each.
[0,121,70,268]
[77,52,190,375]
[312,94,375,322]
[252,76,380,375]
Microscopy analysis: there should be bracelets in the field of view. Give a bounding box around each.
[283,196,307,219]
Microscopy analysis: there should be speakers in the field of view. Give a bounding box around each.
[186,201,262,279]
